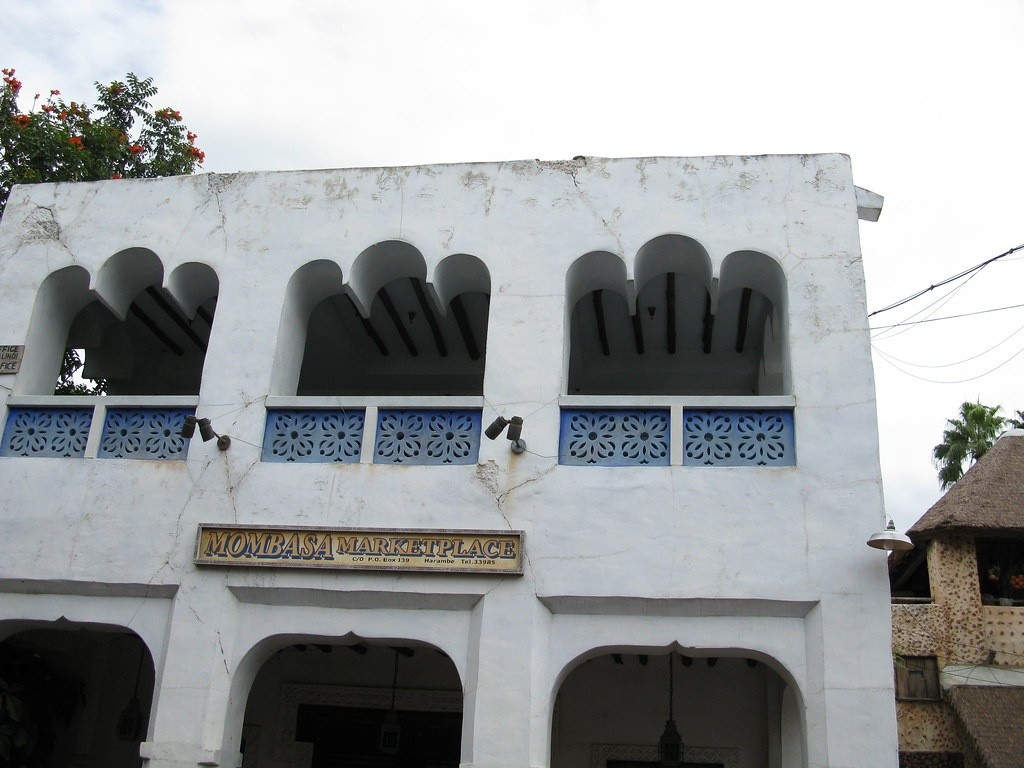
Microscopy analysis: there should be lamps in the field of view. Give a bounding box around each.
[867,519,915,552]
[485,417,527,454]
[378,653,401,755]
[657,654,685,768]
[181,415,231,451]
[117,646,146,742]
